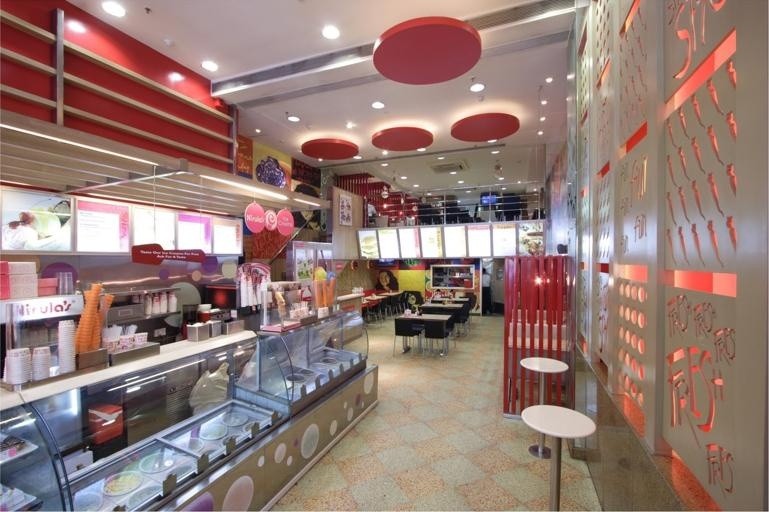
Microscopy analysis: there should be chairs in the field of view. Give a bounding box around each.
[369,192,526,229]
[355,286,480,360]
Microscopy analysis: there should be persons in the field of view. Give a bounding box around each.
[376,270,398,291]
[367,199,376,222]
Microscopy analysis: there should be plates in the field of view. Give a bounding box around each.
[72,410,261,511]
[285,353,353,383]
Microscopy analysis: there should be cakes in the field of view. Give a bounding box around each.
[0,483,25,511]
[0,435,25,461]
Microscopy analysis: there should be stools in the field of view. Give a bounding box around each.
[520,354,571,460]
[517,402,599,511]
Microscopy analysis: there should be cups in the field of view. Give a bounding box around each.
[75,283,114,353]
[290,307,311,320]
[181,302,197,338]
[58,318,77,374]
[131,291,177,318]
[55,271,74,295]
[318,306,330,318]
[4,345,52,386]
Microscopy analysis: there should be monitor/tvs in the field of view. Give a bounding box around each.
[480,194,498,206]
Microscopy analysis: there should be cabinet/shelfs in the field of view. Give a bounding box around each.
[0,306,379,511]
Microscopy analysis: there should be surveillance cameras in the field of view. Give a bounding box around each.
[381,191,388,198]
